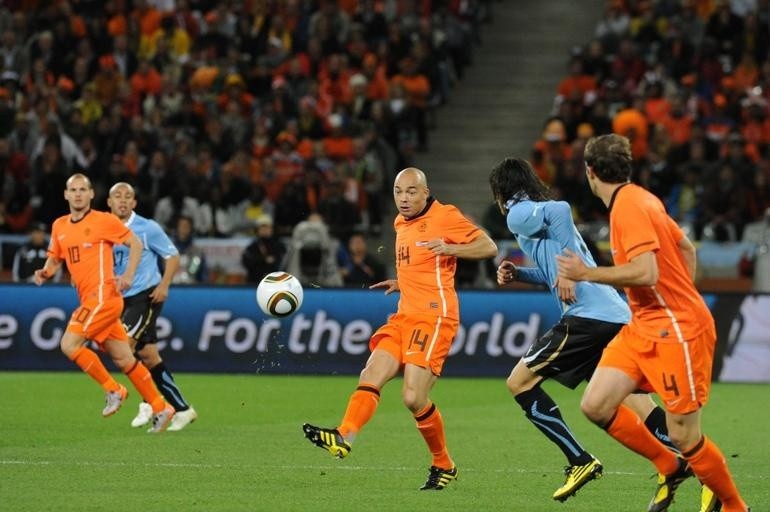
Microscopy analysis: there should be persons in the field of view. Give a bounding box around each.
[488,155,695,505]
[556,131,750,512]
[487,0,770,241]
[34,174,176,434]
[0,0,489,288]
[301,167,500,490]
[106,183,198,432]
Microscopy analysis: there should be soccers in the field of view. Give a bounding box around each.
[257,272,303,317]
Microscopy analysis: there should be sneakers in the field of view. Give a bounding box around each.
[419,462,458,491]
[303,424,352,458]
[648,454,693,512]
[103,384,128,417]
[553,455,603,503]
[131,402,196,433]
[700,483,722,512]
